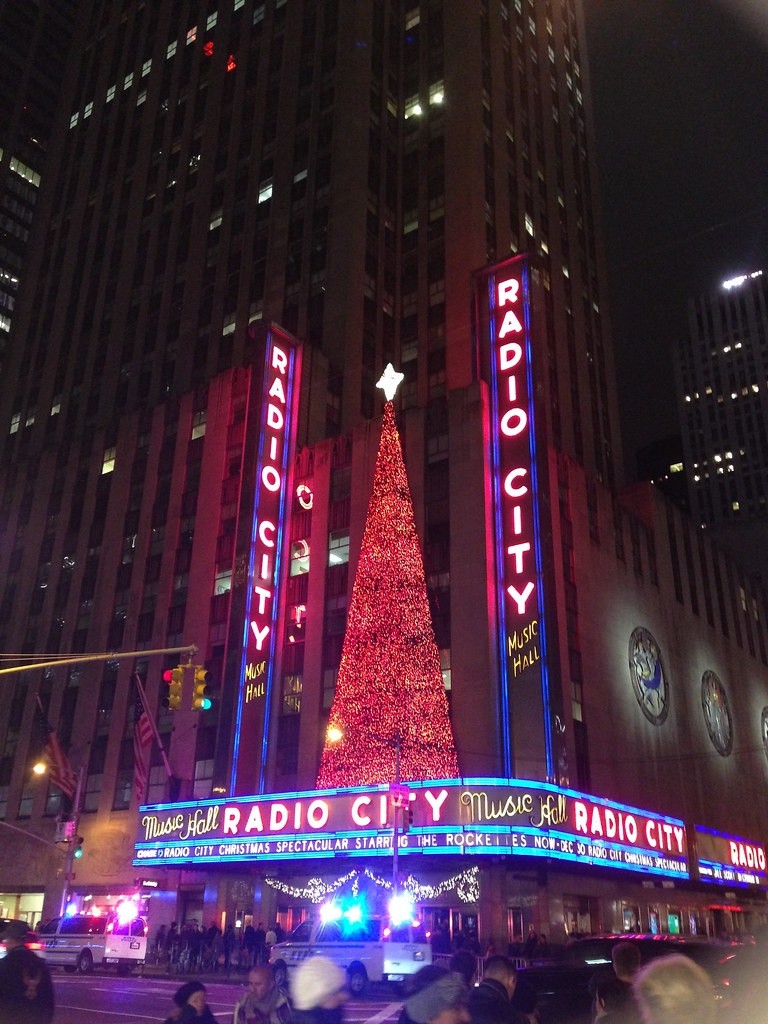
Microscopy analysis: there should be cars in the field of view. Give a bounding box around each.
[0,918,32,958]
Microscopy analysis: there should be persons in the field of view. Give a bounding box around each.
[398,922,768,1024]
[286,956,350,1024]
[233,964,291,1024]
[0,921,54,1024]
[154,917,285,975]
[167,981,218,1024]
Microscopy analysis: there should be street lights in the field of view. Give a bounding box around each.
[328,726,399,896]
[33,762,82,916]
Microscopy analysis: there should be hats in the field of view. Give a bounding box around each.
[178,980,206,1005]
[1,921,40,940]
[405,965,467,1023]
[290,955,347,1011]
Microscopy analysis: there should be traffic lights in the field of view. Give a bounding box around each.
[191,670,212,711]
[161,669,183,711]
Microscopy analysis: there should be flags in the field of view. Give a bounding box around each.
[35,702,79,798]
[131,677,154,806]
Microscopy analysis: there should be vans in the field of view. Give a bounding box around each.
[270,914,432,997]
[38,914,147,975]
